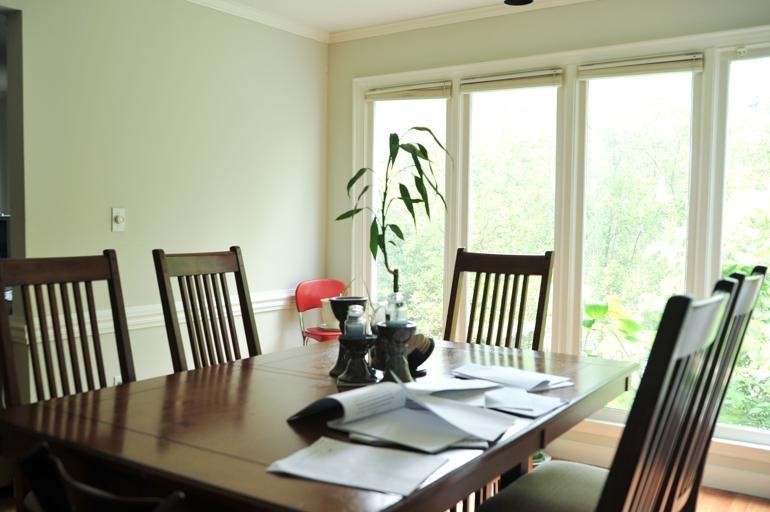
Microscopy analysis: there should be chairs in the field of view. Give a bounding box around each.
[1,250,139,402]
[295,278,349,346]
[475,263,766,508]
[151,245,265,376]
[426,248,556,511]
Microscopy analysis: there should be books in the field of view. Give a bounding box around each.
[284,382,492,453]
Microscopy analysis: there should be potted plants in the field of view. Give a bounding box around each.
[333,126,458,336]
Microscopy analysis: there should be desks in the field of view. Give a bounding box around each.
[0,337,643,512]
